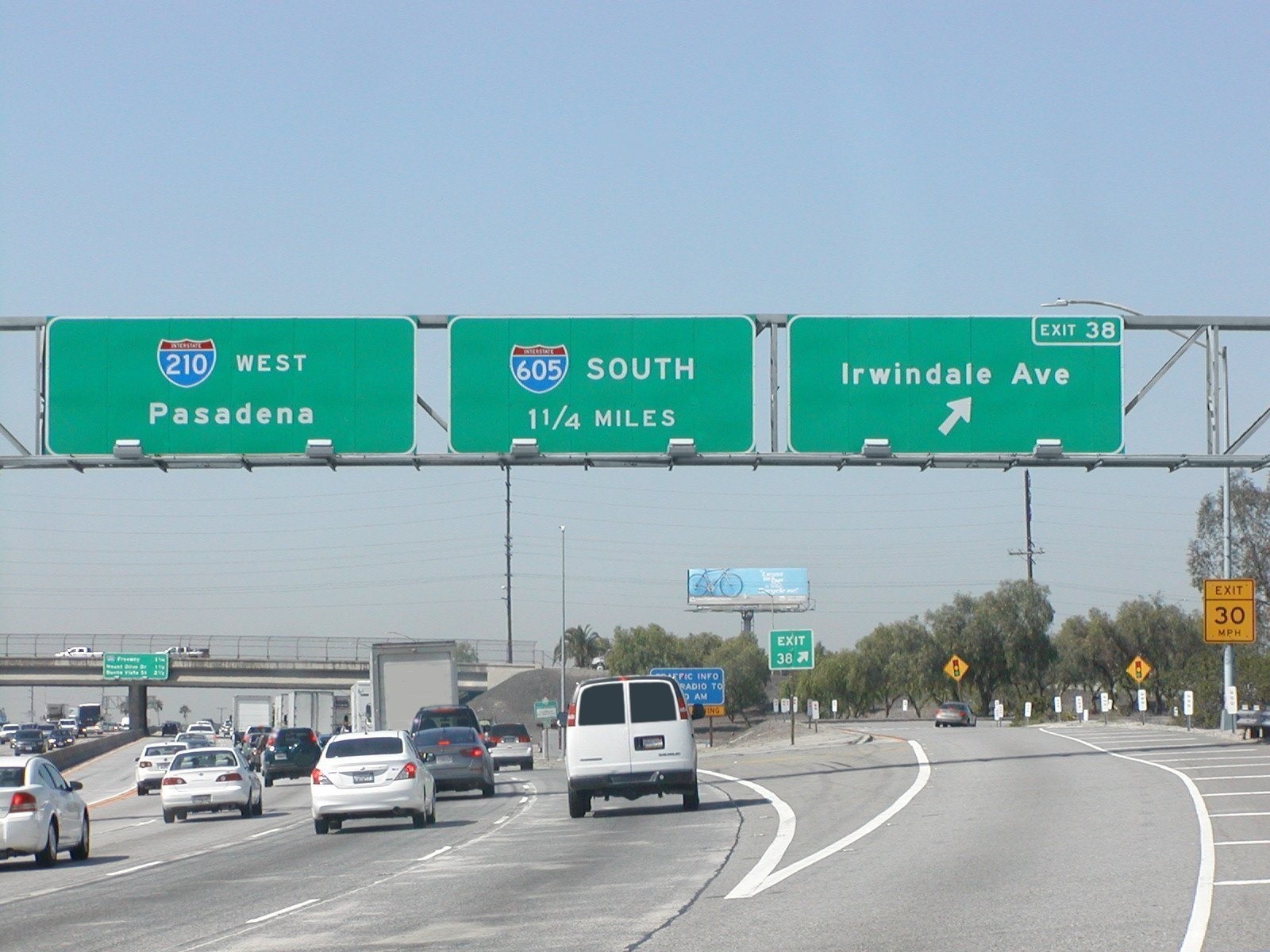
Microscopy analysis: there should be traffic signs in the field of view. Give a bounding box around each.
[102,652,168,681]
[784,314,1124,456]
[649,667,726,717]
[448,316,754,456]
[46,316,418,456]
[533,700,559,722]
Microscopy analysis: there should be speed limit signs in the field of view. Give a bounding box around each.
[769,629,815,671]
[1202,578,1256,645]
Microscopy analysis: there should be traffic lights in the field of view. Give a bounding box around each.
[1125,656,1152,683]
[943,655,969,682]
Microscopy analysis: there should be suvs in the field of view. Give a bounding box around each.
[411,704,491,744]
[556,675,706,818]
[260,728,323,787]
[485,723,533,772]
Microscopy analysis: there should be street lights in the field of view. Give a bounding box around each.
[758,587,774,629]
[216,707,227,725]
[559,525,567,760]
[1042,299,1237,734]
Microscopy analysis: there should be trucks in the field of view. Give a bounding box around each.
[43,702,130,731]
[230,639,458,747]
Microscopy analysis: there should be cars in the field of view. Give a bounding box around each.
[161,719,232,767]
[135,742,198,796]
[311,719,437,834]
[935,701,977,727]
[412,727,497,797]
[240,725,273,774]
[0,718,124,756]
[0,756,90,868]
[592,649,612,670]
[160,747,263,823]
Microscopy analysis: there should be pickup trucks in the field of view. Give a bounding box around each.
[155,647,205,658]
[55,646,104,658]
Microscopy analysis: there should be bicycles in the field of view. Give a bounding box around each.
[688,569,744,597]
